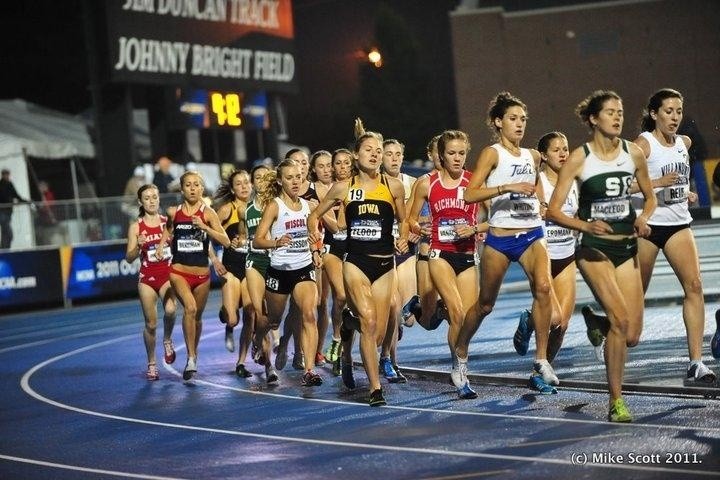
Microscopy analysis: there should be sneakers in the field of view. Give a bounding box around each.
[340,308,354,342]
[183,351,198,380]
[146,362,159,381]
[379,354,408,384]
[683,360,716,386]
[582,305,607,362]
[609,398,631,422]
[367,388,386,406]
[402,294,421,319]
[527,358,560,395]
[513,309,534,355]
[450,346,479,399]
[292,335,356,391]
[162,340,175,364]
[226,325,288,386]
[430,300,447,330]
[710,309,720,358]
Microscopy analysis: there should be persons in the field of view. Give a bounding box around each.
[37,180,56,245]
[120,88,720,422]
[0,169,31,248]
